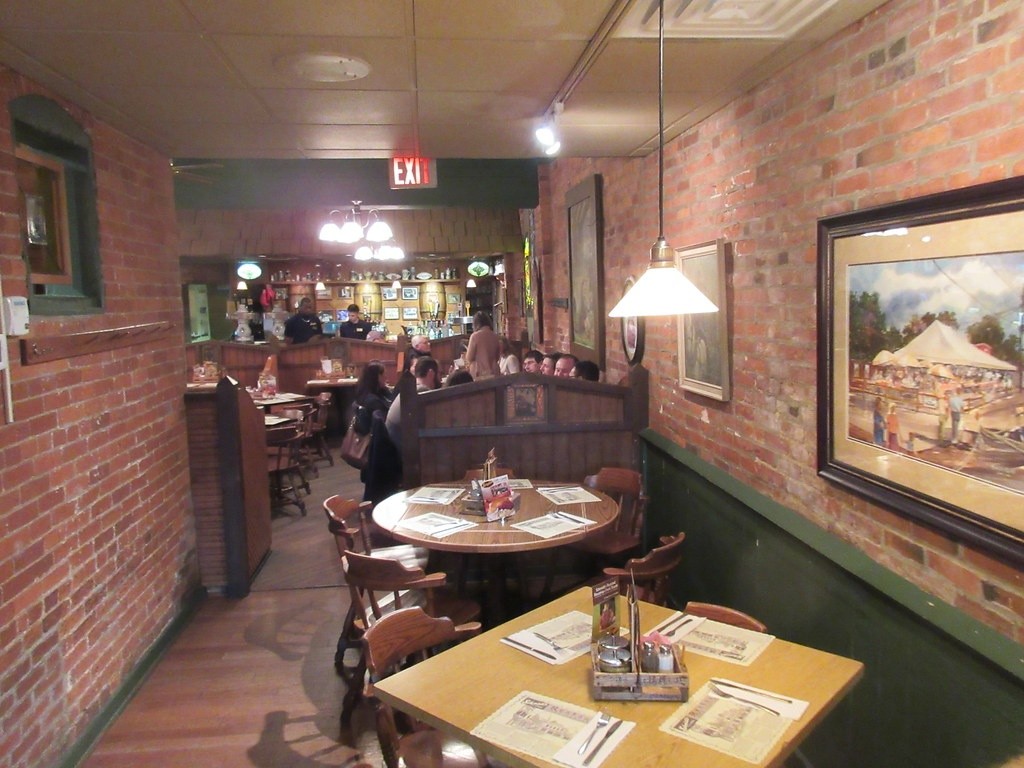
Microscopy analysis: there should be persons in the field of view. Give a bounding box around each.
[448,338,472,373]
[523,350,599,383]
[499,335,520,376]
[385,356,442,453]
[349,361,393,422]
[340,304,372,341]
[283,297,324,345]
[466,311,501,376]
[402,337,432,377]
[445,369,474,389]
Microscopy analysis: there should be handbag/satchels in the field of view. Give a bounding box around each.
[342,416,375,470]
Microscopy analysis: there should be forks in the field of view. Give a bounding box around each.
[534,632,570,650]
[663,619,693,637]
[707,683,780,717]
[434,517,465,526]
[578,713,611,754]
[551,512,579,525]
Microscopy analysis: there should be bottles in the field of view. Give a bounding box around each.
[351,267,356,281]
[315,265,320,281]
[441,266,457,279]
[434,264,439,279]
[658,645,674,673]
[279,270,284,281]
[418,320,452,340]
[233,294,253,313]
[411,267,416,280]
[371,321,387,332]
[347,363,354,378]
[379,272,384,280]
[307,273,311,281]
[318,311,333,322]
[641,642,659,673]
[336,264,341,281]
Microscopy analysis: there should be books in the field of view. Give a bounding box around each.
[477,474,516,522]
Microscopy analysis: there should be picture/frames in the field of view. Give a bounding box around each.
[672,238,730,402]
[402,306,418,320]
[563,172,604,371]
[383,307,400,320]
[401,286,418,300]
[814,176,1024,569]
[335,309,349,323]
[335,286,353,300]
[620,276,645,364]
[381,288,398,300]
[315,309,335,323]
[447,292,461,304]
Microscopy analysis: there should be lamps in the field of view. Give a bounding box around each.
[320,195,405,261]
[610,0,719,317]
[314,266,325,291]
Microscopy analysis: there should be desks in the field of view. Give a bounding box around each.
[373,586,863,768]
[304,377,358,432]
[246,390,315,416]
[372,478,619,632]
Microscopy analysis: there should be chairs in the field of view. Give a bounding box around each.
[257,394,769,768]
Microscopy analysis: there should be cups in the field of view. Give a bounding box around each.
[227,301,236,313]
[331,358,343,379]
[402,270,410,281]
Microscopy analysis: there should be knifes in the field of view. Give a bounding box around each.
[502,636,557,660]
[431,523,469,536]
[709,679,792,703]
[583,720,624,765]
[556,512,584,523]
[649,613,688,636]
